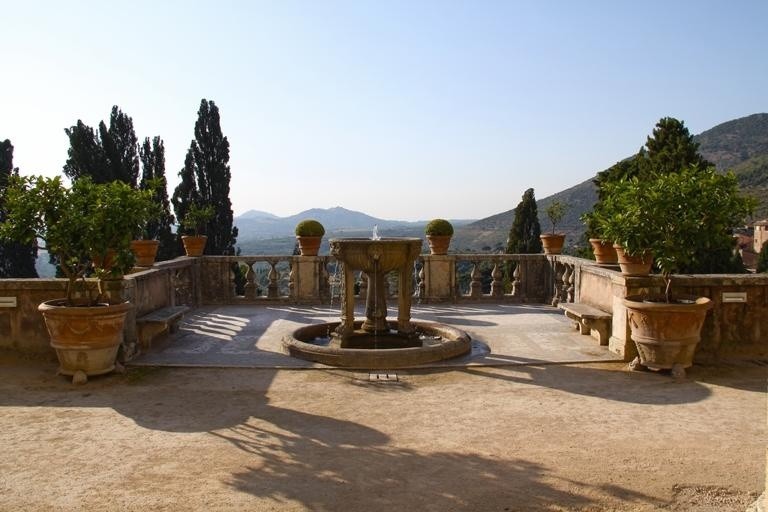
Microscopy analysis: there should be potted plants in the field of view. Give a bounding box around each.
[426,219,454,255]
[579,162,758,372]
[539,197,569,254]
[0,168,167,376]
[295,220,325,256]
[180,201,216,257]
